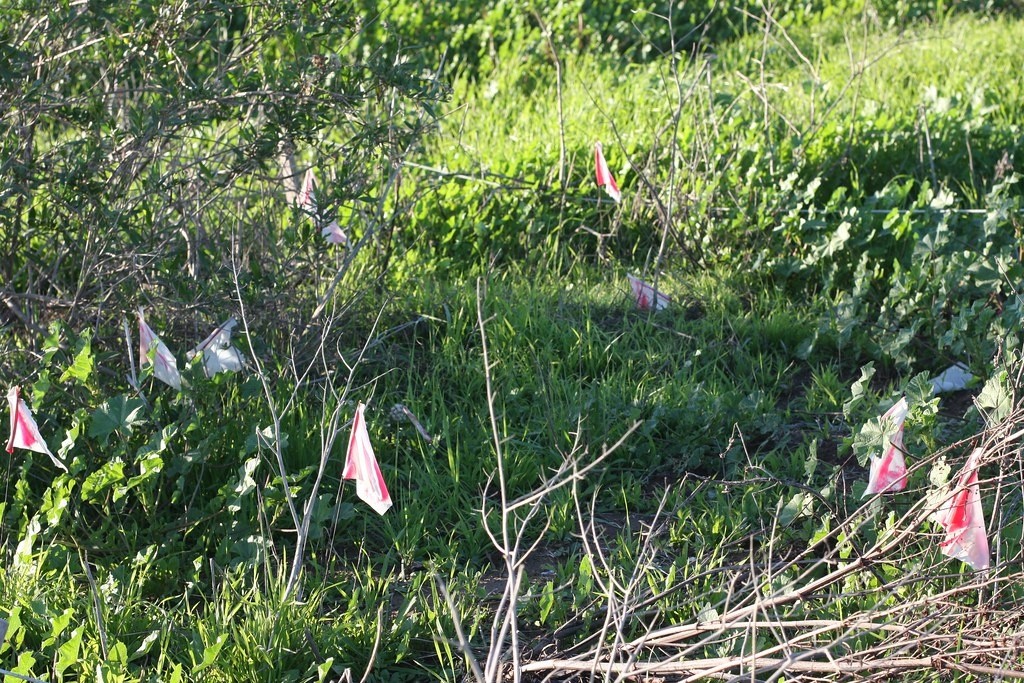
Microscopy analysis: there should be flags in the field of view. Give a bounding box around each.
[187,321,246,381]
[631,277,669,308]
[299,169,345,244]
[6,385,70,473]
[596,142,623,204]
[863,396,991,580]
[137,320,181,388]
[342,409,390,515]
